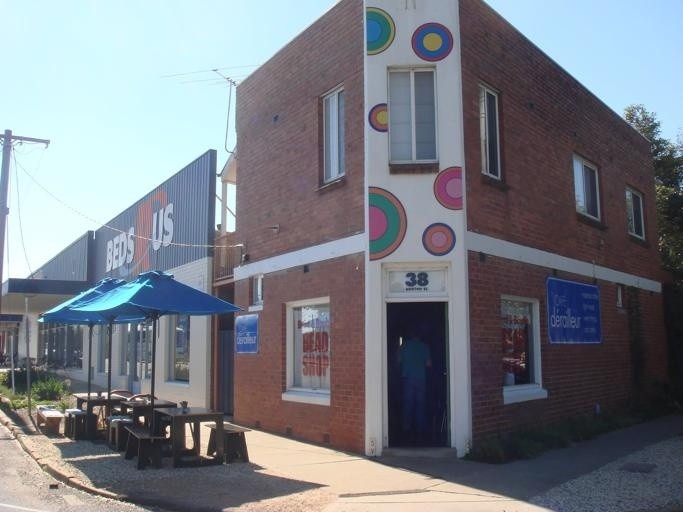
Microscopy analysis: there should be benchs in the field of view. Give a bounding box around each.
[35,404,87,440]
[204,423,251,464]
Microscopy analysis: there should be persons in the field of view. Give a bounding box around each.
[394,326,432,435]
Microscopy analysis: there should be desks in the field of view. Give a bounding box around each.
[71,392,224,470]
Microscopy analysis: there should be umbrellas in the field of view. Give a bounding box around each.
[37,276,147,401]
[67,270,245,432]
